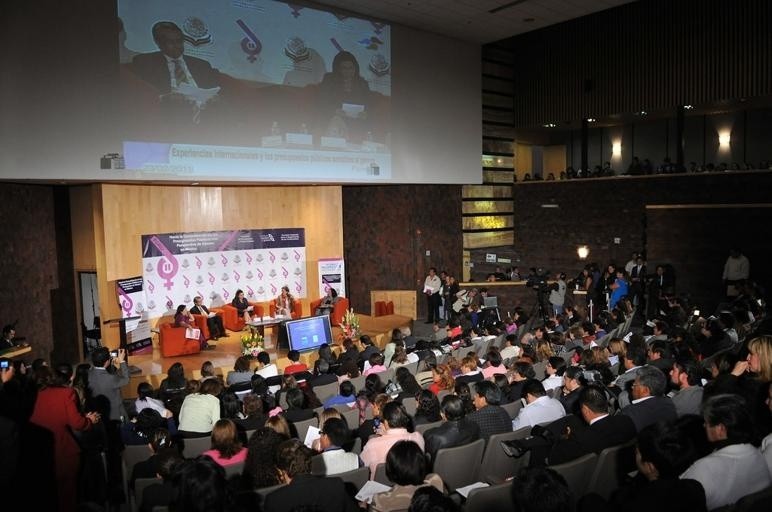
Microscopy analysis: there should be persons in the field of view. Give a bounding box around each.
[311,51,375,145]
[521,158,769,182]
[2,246,771,512]
[132,21,226,147]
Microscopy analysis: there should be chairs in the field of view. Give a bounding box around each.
[94,290,746,510]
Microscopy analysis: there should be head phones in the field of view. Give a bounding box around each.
[561,274,567,279]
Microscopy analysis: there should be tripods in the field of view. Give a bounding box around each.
[527,289,548,332]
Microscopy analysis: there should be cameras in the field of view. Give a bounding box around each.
[109,350,118,357]
[0,358,12,369]
[603,287,613,293]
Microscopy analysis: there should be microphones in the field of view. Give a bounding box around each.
[119,304,123,318]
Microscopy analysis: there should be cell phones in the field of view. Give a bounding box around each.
[373,416,381,434]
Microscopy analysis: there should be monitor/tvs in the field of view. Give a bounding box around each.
[285,315,333,353]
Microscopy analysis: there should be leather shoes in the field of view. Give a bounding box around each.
[500,439,527,457]
